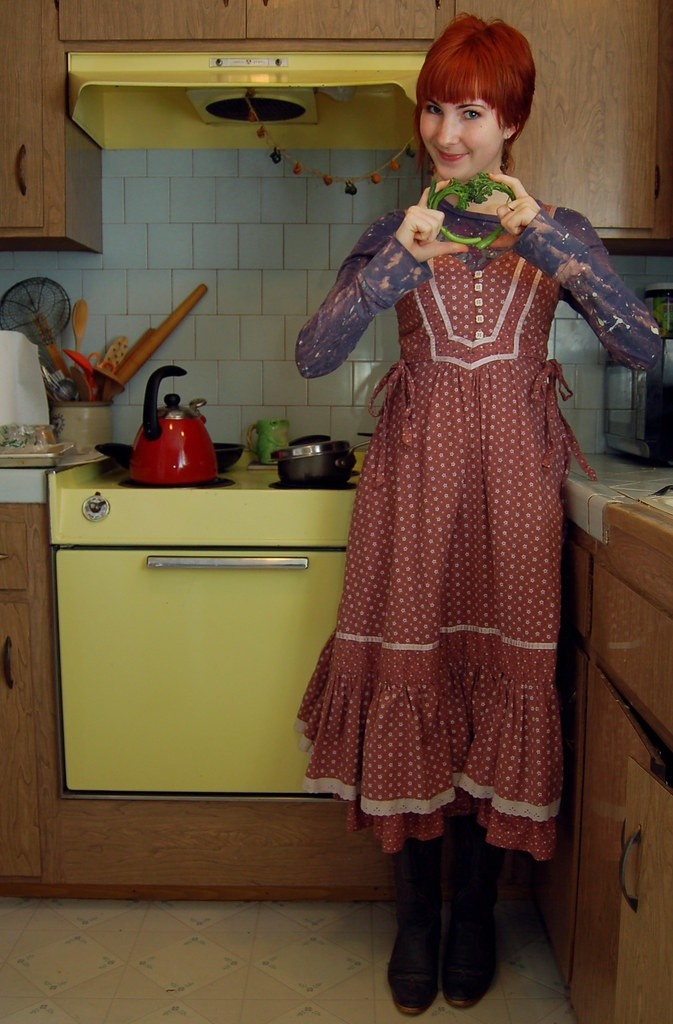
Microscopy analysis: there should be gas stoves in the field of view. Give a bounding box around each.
[49,466,362,546]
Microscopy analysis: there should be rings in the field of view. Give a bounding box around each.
[507,202,515,211]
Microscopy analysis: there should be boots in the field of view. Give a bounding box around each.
[440,813,505,1007]
[387,835,442,1014]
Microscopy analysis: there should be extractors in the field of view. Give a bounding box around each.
[66,51,427,152]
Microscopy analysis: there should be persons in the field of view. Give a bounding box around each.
[294,12,663,1014]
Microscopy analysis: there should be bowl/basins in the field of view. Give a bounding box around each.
[50,401,114,454]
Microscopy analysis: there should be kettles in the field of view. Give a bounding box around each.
[130,367,217,485]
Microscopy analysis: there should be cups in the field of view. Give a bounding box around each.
[247,420,290,464]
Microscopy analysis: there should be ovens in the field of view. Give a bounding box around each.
[56,547,346,792]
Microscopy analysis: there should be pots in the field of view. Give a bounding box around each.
[95,442,245,470]
[269,435,371,485]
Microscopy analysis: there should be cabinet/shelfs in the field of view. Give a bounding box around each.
[534,520,673,1024]
[454,0,673,238]
[0,504,59,877]
[58,0,437,41]
[0,0,102,254]
